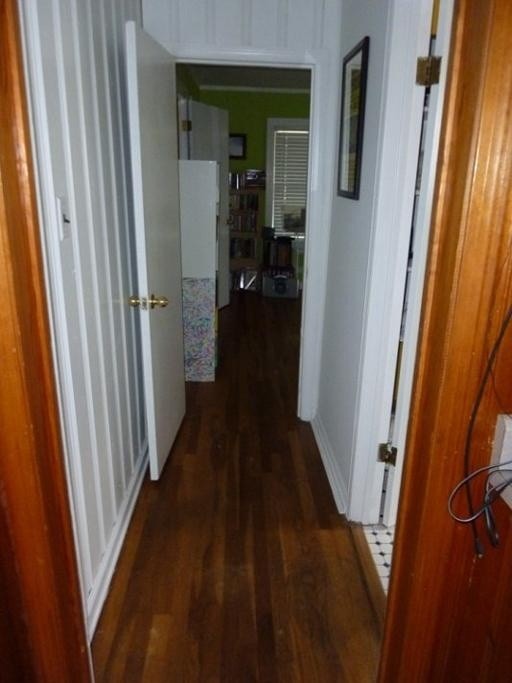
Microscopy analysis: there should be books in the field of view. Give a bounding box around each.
[227,169,266,288]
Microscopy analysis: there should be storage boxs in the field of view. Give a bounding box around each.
[263,277,300,299]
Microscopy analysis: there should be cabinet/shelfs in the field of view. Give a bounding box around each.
[229,186,266,270]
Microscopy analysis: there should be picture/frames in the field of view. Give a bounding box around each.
[337,33,371,199]
[228,132,247,160]
